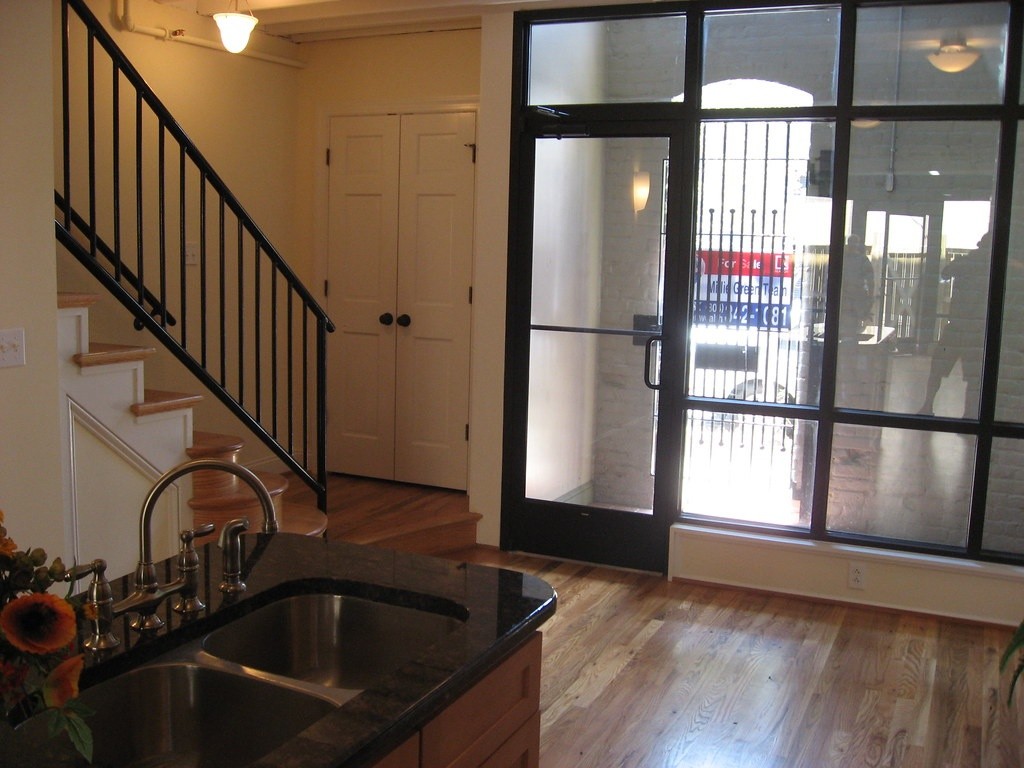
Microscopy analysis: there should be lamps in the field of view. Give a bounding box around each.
[634,171,650,211]
[213,0,258,54]
[928,36,980,73]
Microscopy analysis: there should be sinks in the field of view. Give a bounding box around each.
[191,578,471,696]
[0,656,348,768]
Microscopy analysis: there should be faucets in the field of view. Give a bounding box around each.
[130,458,278,634]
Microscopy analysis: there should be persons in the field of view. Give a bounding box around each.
[913,228,990,430]
[838,234,874,338]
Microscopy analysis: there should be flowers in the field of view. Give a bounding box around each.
[0,510,98,764]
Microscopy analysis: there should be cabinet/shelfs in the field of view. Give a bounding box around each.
[369,631,543,768]
